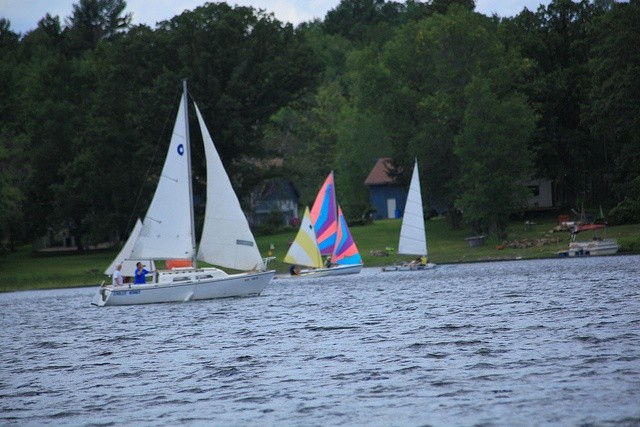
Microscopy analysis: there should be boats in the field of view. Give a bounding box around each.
[568,223,621,256]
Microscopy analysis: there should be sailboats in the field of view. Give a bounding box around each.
[283,168,365,279]
[381,158,439,270]
[90,78,277,308]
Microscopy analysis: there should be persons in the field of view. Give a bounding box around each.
[324,257,332,268]
[134,262,156,284]
[290,265,296,275]
[407,257,422,268]
[113,263,123,287]
